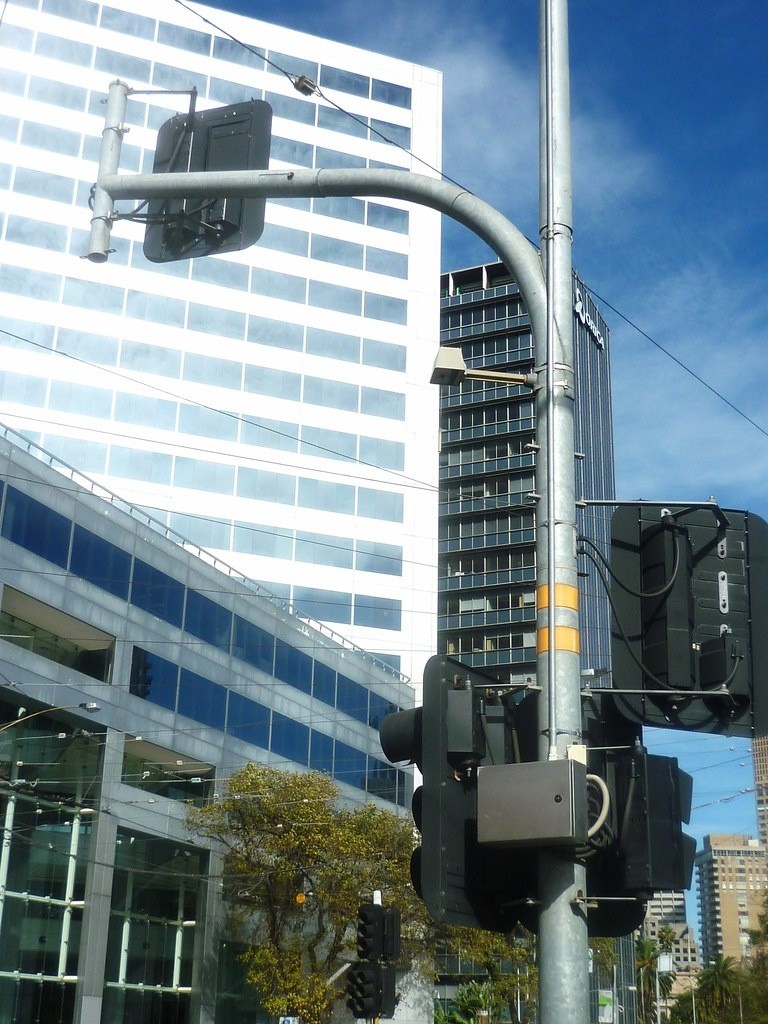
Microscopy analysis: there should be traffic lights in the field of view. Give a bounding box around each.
[357,903,387,959]
[347,960,367,1017]
[358,960,381,1018]
[378,652,510,934]
[646,743,698,896]
